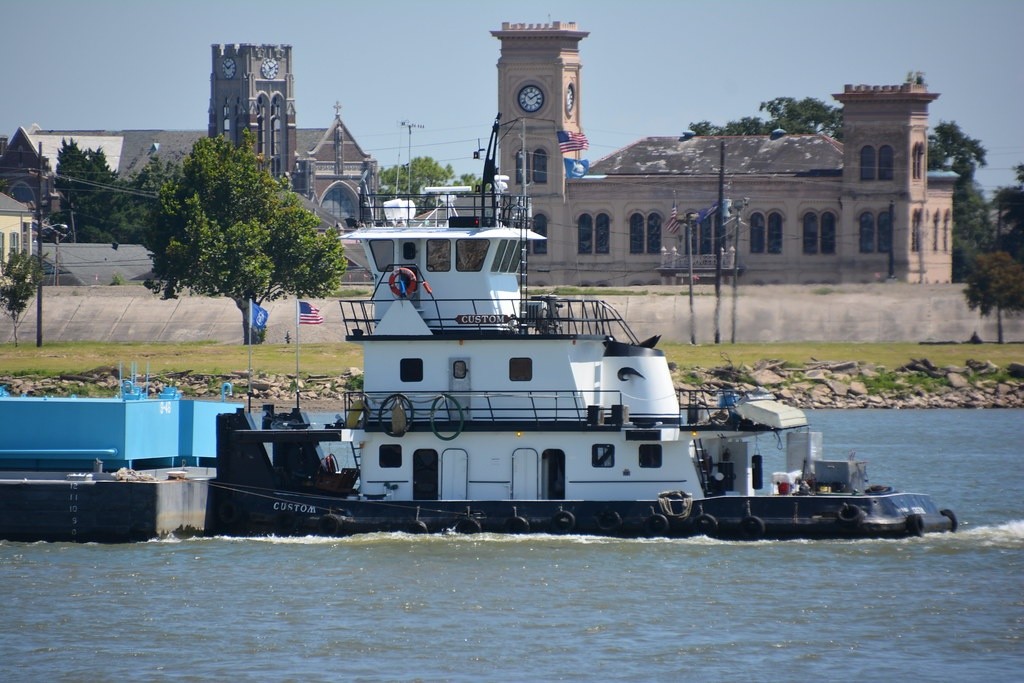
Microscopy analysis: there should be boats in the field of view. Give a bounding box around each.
[214,120,958,540]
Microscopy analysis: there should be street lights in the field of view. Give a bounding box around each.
[38,223,68,347]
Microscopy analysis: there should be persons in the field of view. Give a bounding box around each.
[971,332,982,343]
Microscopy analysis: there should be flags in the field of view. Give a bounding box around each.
[249,301,269,330]
[297,300,324,324]
[565,158,589,179]
[697,201,720,224]
[557,130,589,151]
[666,203,681,234]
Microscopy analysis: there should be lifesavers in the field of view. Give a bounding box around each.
[388,266,419,297]
[451,517,482,533]
[940,509,959,533]
[642,513,670,537]
[907,514,925,537]
[738,516,765,540]
[837,503,864,523]
[400,520,430,534]
[502,516,531,534]
[318,513,344,537]
[691,513,719,537]
[550,511,576,533]
[326,456,336,474]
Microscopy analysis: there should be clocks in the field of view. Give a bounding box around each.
[222,58,236,80]
[262,59,279,79]
[518,86,544,112]
[567,87,574,111]
